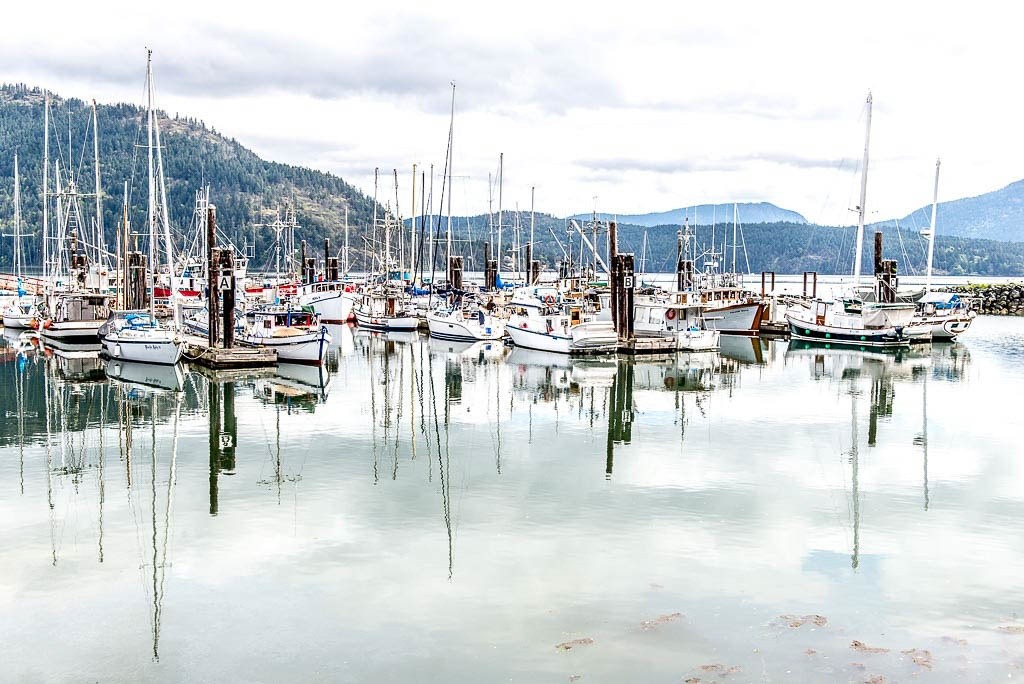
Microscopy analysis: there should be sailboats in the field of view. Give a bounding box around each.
[0,47,434,366]
[428,80,975,355]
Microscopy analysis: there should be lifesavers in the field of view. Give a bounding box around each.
[545,295,555,307]
[666,308,677,320]
